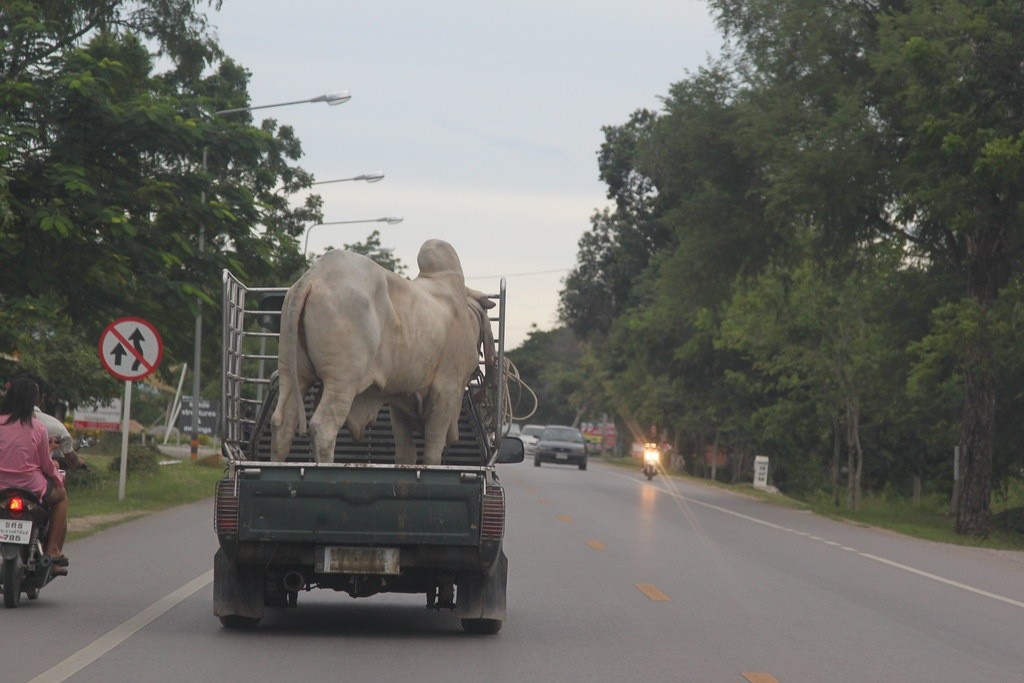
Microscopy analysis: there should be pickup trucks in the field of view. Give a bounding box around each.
[214,268,526,635]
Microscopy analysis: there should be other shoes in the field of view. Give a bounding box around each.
[53,569,67,576]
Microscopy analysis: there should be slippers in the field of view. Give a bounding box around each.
[51,553,70,567]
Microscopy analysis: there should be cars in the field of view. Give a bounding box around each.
[533,425,591,470]
[499,422,520,438]
[518,425,546,457]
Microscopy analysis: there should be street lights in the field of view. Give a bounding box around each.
[272,173,386,232]
[190,88,352,466]
[304,218,404,262]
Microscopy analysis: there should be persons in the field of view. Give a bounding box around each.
[0,378,69,566]
[34,382,85,576]
[640,425,661,473]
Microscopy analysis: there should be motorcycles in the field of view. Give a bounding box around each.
[0,434,97,608]
[644,449,659,481]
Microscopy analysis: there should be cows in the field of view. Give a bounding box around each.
[266,238,496,465]
